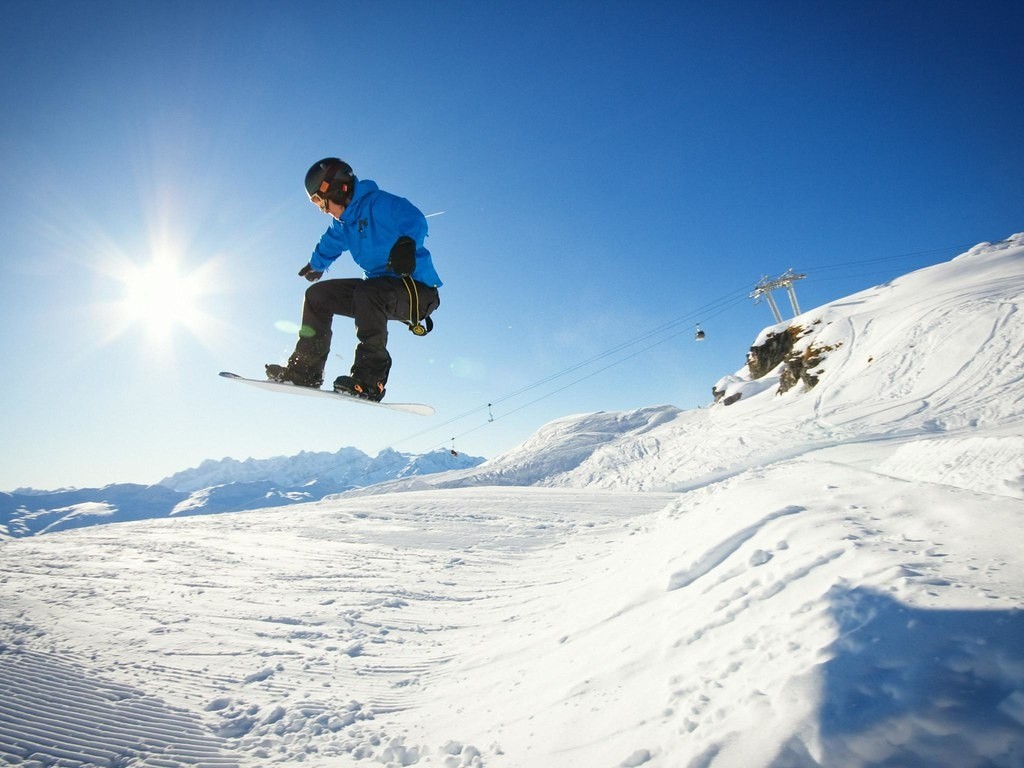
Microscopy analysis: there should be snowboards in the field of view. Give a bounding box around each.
[219,371,435,416]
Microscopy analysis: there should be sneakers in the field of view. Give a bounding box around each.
[265,363,322,387]
[334,370,384,401]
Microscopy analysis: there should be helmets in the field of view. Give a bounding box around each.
[305,158,354,203]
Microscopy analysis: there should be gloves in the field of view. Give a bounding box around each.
[298,263,323,282]
[384,236,416,276]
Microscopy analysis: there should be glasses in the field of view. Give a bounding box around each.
[310,191,328,213]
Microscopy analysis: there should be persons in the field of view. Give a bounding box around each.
[265,156,443,403]
